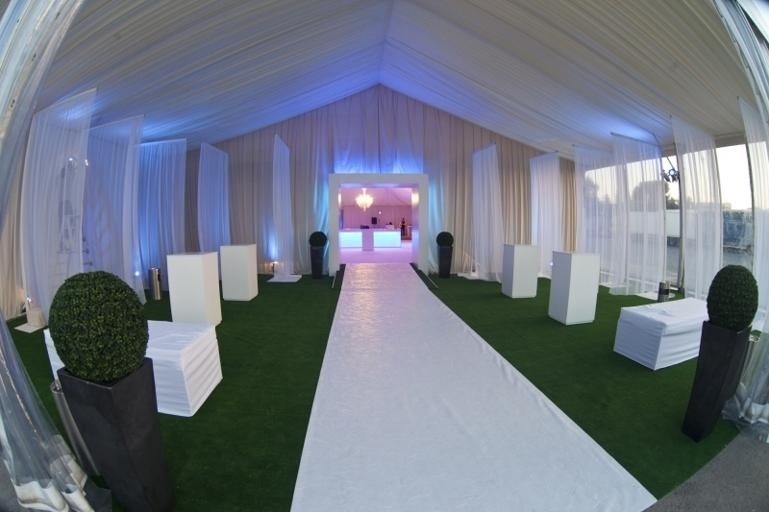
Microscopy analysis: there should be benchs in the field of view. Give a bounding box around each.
[41,316,224,418]
[612,296,709,372]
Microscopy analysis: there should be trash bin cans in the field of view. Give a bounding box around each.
[659,283,669,302]
[149,268,161,300]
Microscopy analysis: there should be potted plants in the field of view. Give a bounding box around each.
[309,231,330,278]
[436,231,454,278]
[680,264,760,444]
[46,269,175,511]
